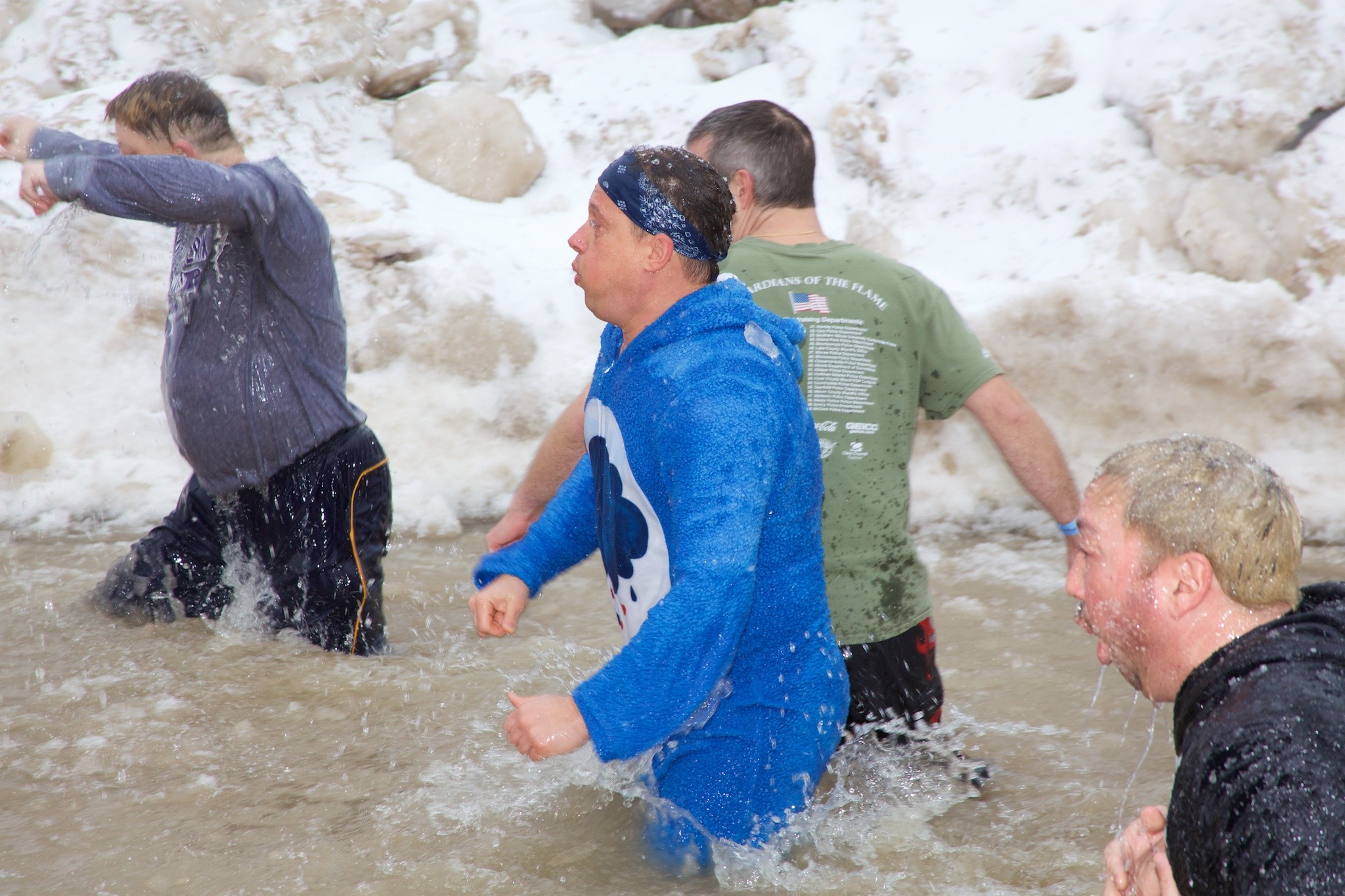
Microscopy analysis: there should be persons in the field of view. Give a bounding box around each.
[484,100,1082,733]
[0,70,393,657]
[1065,435,1345,896]
[467,144,849,848]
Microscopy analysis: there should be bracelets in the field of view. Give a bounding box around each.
[1057,520,1080,536]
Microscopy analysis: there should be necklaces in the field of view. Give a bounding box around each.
[751,230,821,237]
